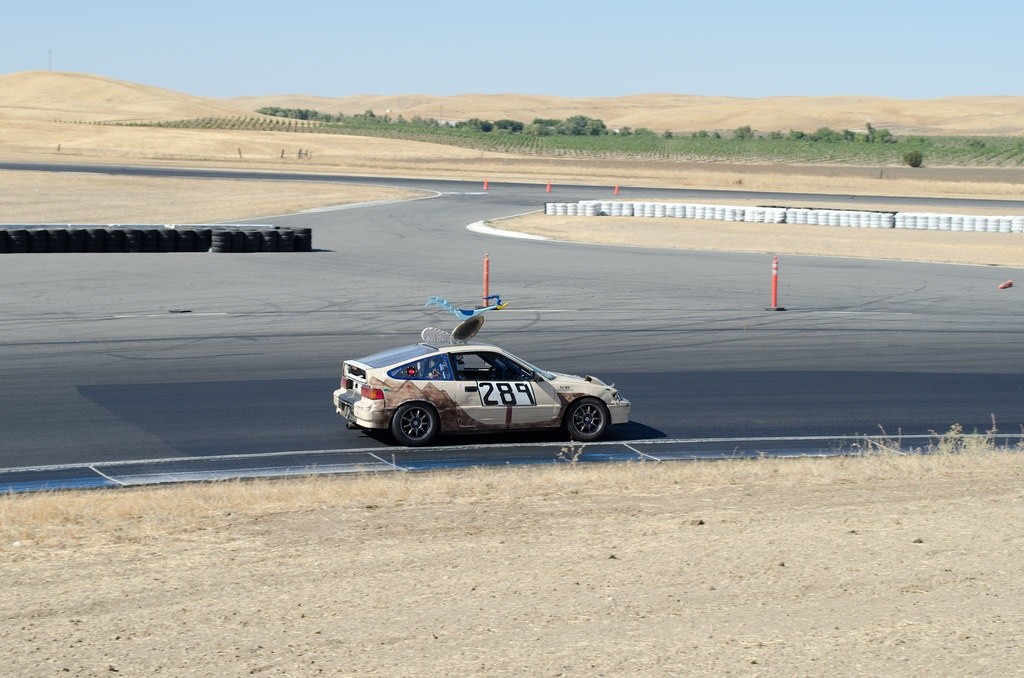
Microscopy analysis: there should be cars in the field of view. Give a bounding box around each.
[334,336,632,448]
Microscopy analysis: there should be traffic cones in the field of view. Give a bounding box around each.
[613,183,621,195]
[997,279,1015,289]
[546,180,552,192]
[483,179,490,190]
